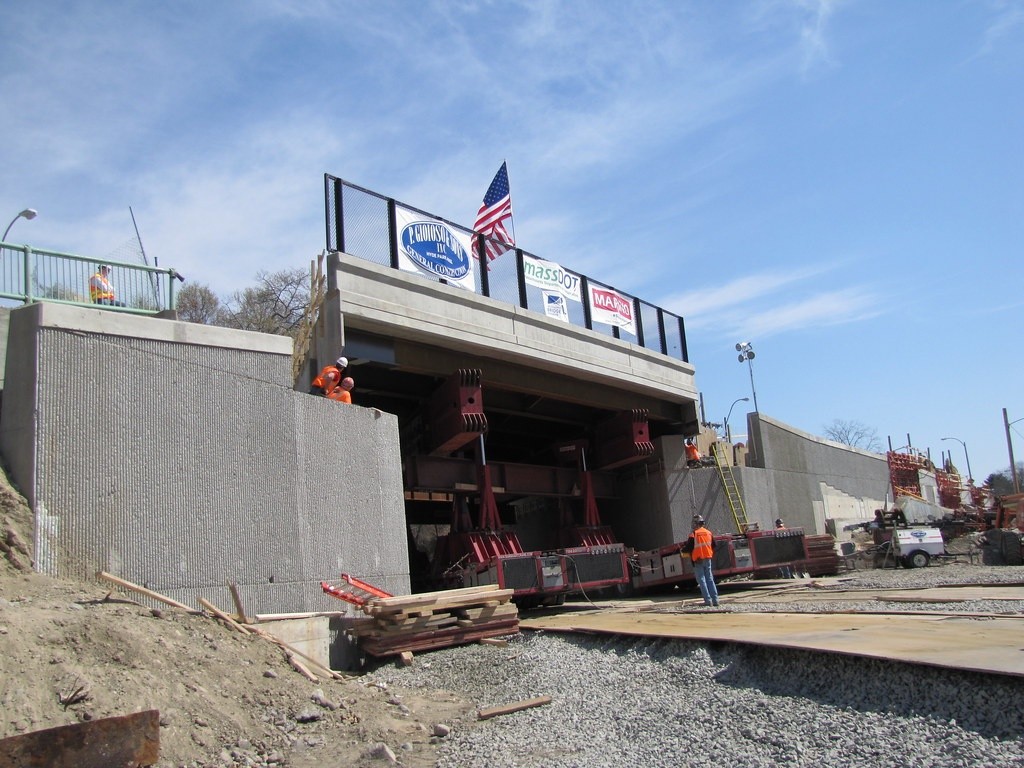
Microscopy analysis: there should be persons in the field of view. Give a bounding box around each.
[309,357,348,397]
[687,514,721,607]
[774,519,787,530]
[89,263,127,307]
[684,434,703,468]
[326,377,354,404]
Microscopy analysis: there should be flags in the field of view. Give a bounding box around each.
[471,163,515,269]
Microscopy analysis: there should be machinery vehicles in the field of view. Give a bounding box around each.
[403,366,808,611]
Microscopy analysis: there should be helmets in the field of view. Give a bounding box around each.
[692,515,706,522]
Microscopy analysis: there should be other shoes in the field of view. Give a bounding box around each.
[713,604,720,606]
[699,603,713,607]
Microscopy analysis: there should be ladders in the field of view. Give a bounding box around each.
[319,572,397,607]
[711,443,750,534]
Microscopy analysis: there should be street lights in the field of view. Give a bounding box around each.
[941,435,973,482]
[723,397,749,441]
[736,341,758,413]
